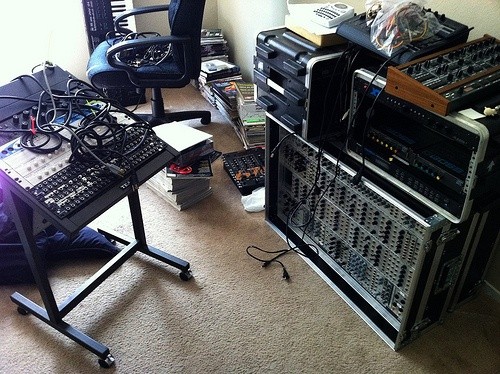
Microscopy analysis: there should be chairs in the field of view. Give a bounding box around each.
[87,0,211,126]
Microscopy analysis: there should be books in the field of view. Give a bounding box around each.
[144,150,222,211]
[189,28,266,152]
